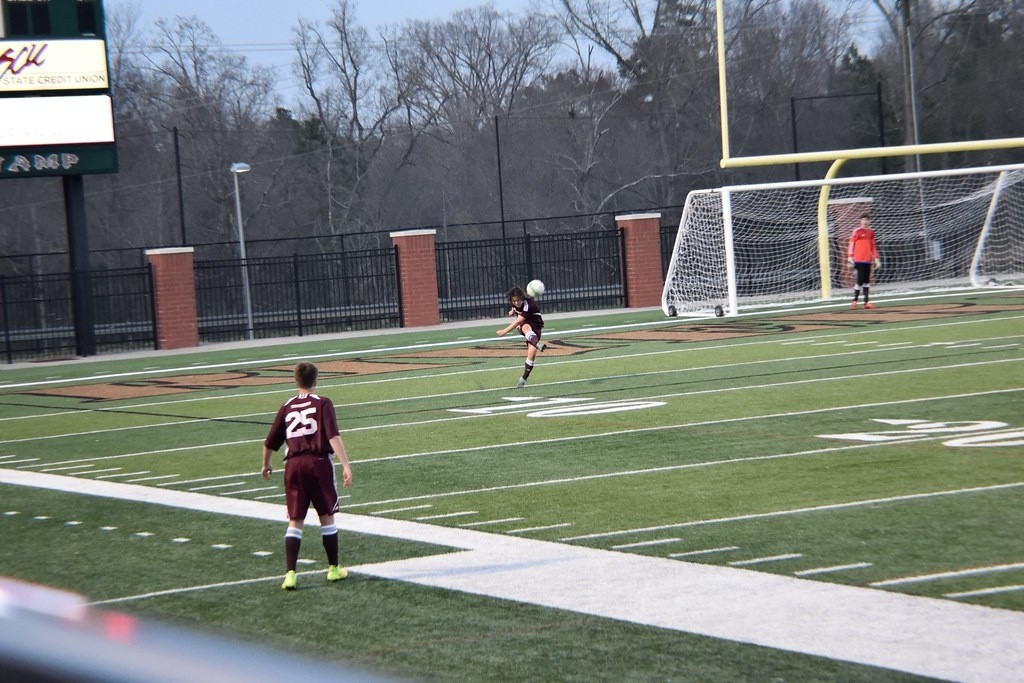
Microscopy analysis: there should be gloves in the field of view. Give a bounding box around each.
[848,257,855,267]
[875,258,881,271]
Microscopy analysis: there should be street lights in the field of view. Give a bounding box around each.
[231,162,254,340]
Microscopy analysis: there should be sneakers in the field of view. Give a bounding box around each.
[327,565,348,581]
[536,340,546,352]
[864,303,873,309]
[517,381,524,389]
[281,570,297,589]
[851,301,857,309]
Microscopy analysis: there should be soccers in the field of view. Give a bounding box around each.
[526,280,545,297]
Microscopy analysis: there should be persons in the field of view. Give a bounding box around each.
[496,287,547,388]
[846,212,881,310]
[261,363,354,589]
